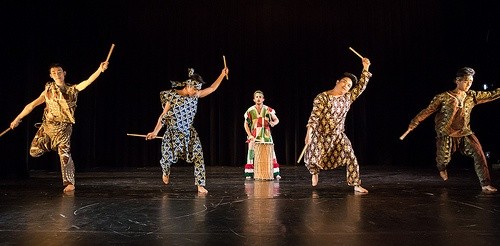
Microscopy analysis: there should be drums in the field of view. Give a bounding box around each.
[253,142,273,183]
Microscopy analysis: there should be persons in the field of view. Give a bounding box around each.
[9,62,109,192]
[409,67,500,191]
[244,91,281,180]
[305,58,372,192]
[146,68,228,193]
